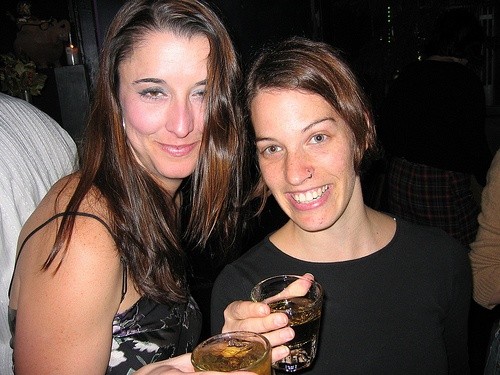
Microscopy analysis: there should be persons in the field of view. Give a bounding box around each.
[0,89,84,375]
[209,38,474,375]
[470,148,500,375]
[382,5,493,259]
[8,0,255,375]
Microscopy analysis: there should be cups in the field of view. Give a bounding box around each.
[191,331,272,375]
[250,275,323,372]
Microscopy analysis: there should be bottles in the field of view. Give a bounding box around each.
[64,32,79,65]
